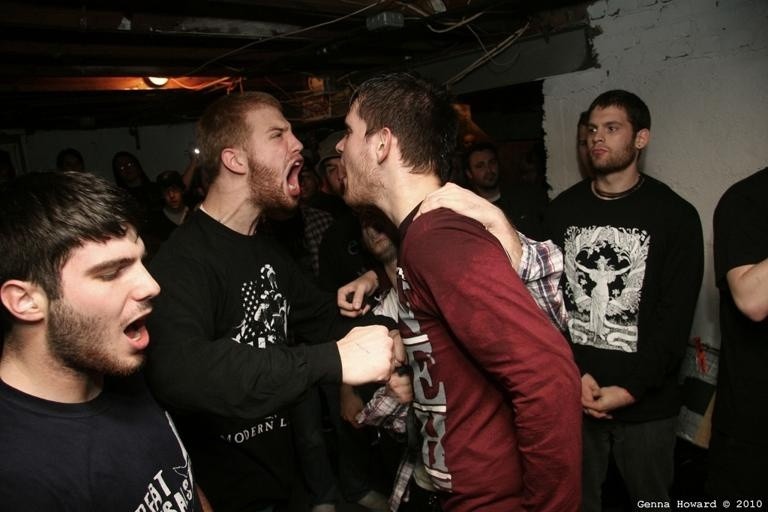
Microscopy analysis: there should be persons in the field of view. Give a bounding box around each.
[708,167,768,511]
[1,71,581,512]
[546,90,705,511]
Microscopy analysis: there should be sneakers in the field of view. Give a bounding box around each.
[358,489,390,512]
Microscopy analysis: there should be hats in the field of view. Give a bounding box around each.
[317,129,346,176]
